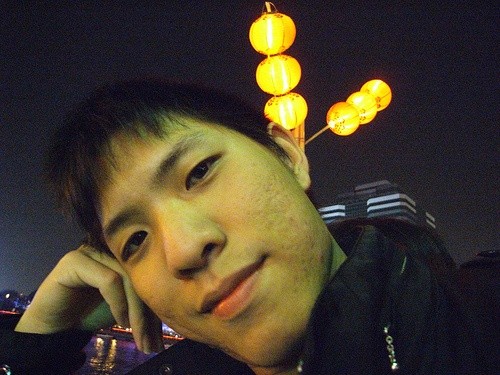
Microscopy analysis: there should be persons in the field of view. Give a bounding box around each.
[0,78,500,375]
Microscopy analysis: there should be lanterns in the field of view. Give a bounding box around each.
[325,78,392,136]
[249,2,309,131]
